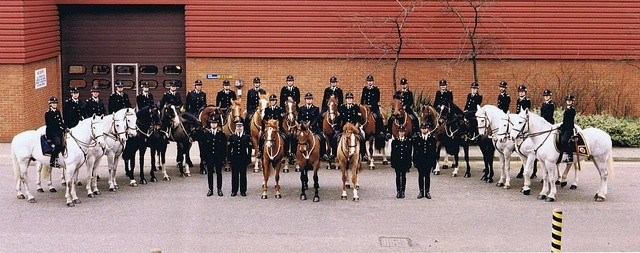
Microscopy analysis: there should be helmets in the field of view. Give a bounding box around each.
[439,79,447,86]
[305,92,313,99]
[253,77,261,83]
[48,97,58,104]
[398,125,407,131]
[141,81,149,87]
[543,89,552,96]
[209,113,219,122]
[286,75,294,81]
[195,80,202,86]
[345,93,353,99]
[115,81,124,87]
[518,85,527,92]
[330,77,337,82]
[236,121,243,127]
[499,81,507,88]
[421,122,429,129]
[70,86,80,93]
[90,86,100,93]
[566,95,574,101]
[223,80,230,86]
[400,78,408,84]
[169,81,178,87]
[270,94,277,100]
[367,75,374,81]
[470,82,479,88]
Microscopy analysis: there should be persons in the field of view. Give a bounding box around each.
[108,81,132,114]
[280,75,300,113]
[540,89,556,125]
[45,96,70,167]
[293,93,326,157]
[225,120,253,196]
[497,81,511,114]
[159,81,183,115]
[244,77,266,134]
[464,82,483,141]
[386,78,420,139]
[321,76,343,112]
[433,80,453,116]
[331,91,367,158]
[256,94,290,158]
[62,87,88,129]
[360,74,386,137]
[185,79,207,119]
[515,85,531,114]
[215,79,237,125]
[412,122,437,199]
[200,113,227,196]
[556,95,576,163]
[136,82,154,110]
[390,126,412,198]
[84,86,107,117]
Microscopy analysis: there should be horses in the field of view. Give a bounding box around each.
[148,102,184,182]
[223,97,242,172]
[418,102,455,174]
[11,113,107,207]
[166,105,202,177]
[389,96,413,138]
[36,109,129,193]
[248,92,270,173]
[510,106,614,202]
[475,104,516,188]
[321,93,343,170]
[445,103,496,183]
[498,109,538,194]
[356,104,388,169]
[198,107,227,132]
[76,107,138,197]
[262,118,287,199]
[279,96,302,173]
[336,118,362,202]
[122,104,164,186]
[293,120,322,202]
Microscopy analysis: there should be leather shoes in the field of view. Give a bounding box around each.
[241,192,246,196]
[231,192,237,196]
[207,189,213,196]
[397,191,405,199]
[50,163,61,167]
[218,190,223,196]
[417,192,424,199]
[425,192,431,199]
